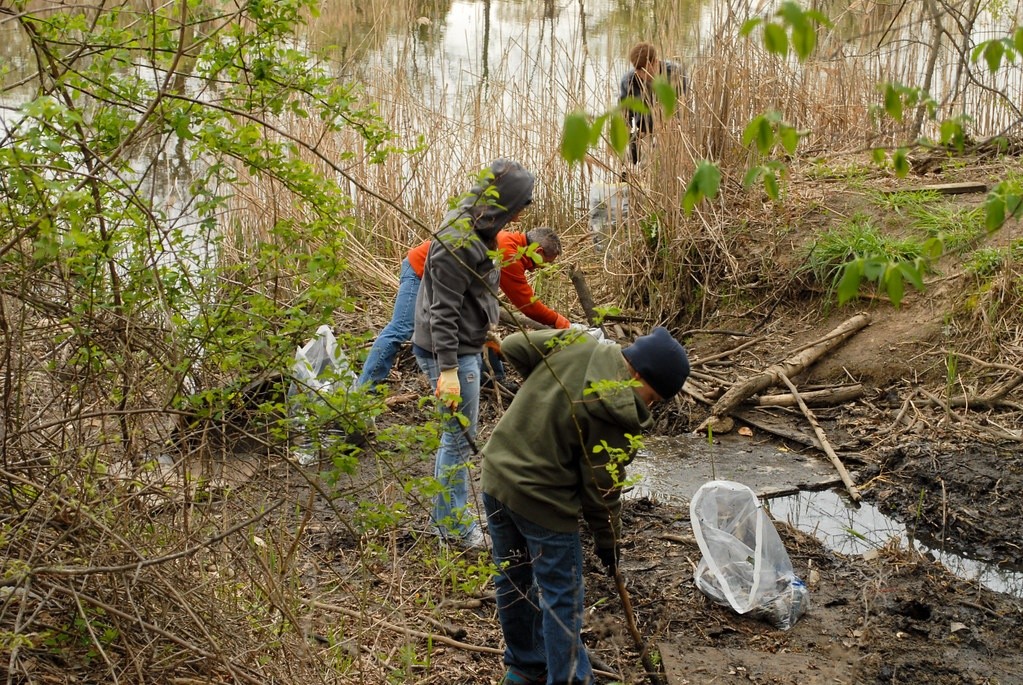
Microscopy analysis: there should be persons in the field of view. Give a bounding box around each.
[409,155,534,549]
[478,327,691,684]
[620,42,687,180]
[357,227,571,394]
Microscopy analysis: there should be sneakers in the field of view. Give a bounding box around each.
[441,526,494,550]
[502,665,547,685]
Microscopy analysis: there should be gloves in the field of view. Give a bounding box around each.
[593,546,619,577]
[434,367,460,412]
[555,313,571,329]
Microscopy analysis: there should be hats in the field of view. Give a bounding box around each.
[621,327,690,401]
